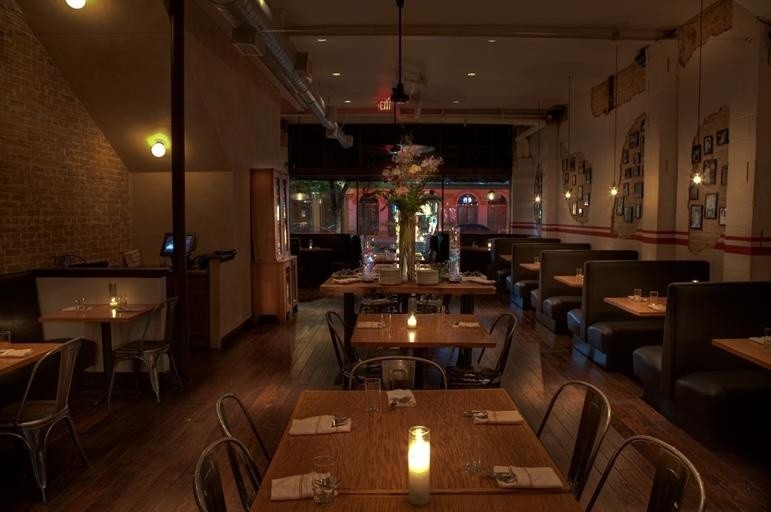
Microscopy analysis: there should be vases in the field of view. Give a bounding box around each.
[398,220,415,283]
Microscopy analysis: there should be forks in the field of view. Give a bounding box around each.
[312,397,516,486]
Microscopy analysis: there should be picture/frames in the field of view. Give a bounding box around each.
[532,170,545,226]
[561,156,593,217]
[612,116,644,221]
[690,126,729,231]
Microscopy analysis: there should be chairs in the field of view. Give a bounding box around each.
[215,393,273,482]
[193,436,262,511]
[537,381,612,503]
[0,290,183,502]
[584,436,706,511]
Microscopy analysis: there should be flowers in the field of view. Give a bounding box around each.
[367,135,444,282]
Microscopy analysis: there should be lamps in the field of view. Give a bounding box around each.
[610,44,618,194]
[390,0,409,105]
[150,141,166,158]
[692,0,701,186]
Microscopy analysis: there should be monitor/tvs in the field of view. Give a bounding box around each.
[160,232,197,256]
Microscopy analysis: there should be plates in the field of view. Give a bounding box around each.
[378,267,402,285]
[416,269,439,286]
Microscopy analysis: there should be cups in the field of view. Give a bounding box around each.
[364,374,382,414]
[649,290,658,304]
[0,331,10,353]
[534,256,539,265]
[632,288,641,303]
[377,312,391,338]
[765,327,771,352]
[309,455,338,503]
[575,267,582,282]
[407,298,419,329]
[437,305,446,320]
[69,281,128,312]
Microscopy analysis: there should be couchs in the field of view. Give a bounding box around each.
[632,282,770,446]
[568,259,710,371]
[290,233,361,290]
[459,233,640,334]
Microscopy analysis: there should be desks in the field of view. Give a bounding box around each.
[712,336,771,370]
[249,246,583,393]
[604,294,669,317]
[252,389,582,510]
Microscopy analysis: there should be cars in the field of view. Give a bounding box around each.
[448,223,494,233]
[290,221,334,234]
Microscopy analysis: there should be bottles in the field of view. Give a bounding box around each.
[407,425,433,508]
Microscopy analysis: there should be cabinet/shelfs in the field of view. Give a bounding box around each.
[249,168,299,326]
